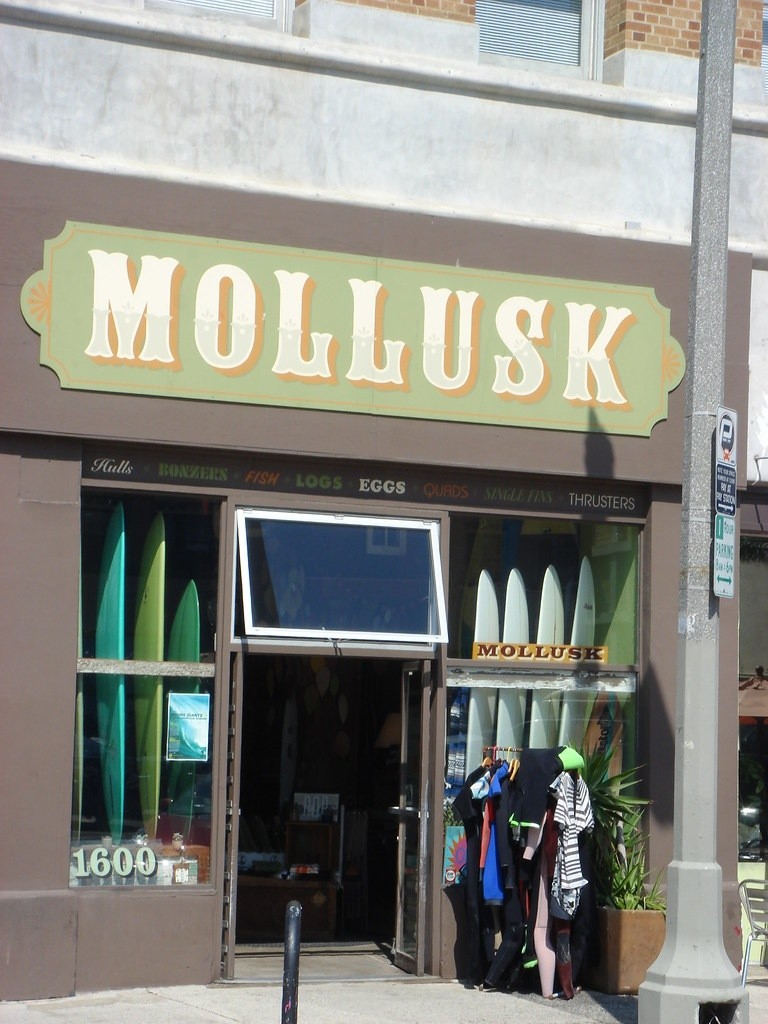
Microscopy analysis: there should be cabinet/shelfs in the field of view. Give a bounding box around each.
[287,821,339,871]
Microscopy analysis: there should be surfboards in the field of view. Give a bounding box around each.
[131,504,168,842]
[164,577,207,842]
[465,554,601,783]
[96,503,129,840]
[73,511,86,839]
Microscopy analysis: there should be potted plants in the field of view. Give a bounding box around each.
[284,801,303,821]
[102,834,112,850]
[320,804,337,822]
[581,840,666,994]
[171,833,184,851]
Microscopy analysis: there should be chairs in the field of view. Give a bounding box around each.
[737,879,768,988]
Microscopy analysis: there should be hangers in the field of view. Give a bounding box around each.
[480,745,579,782]
